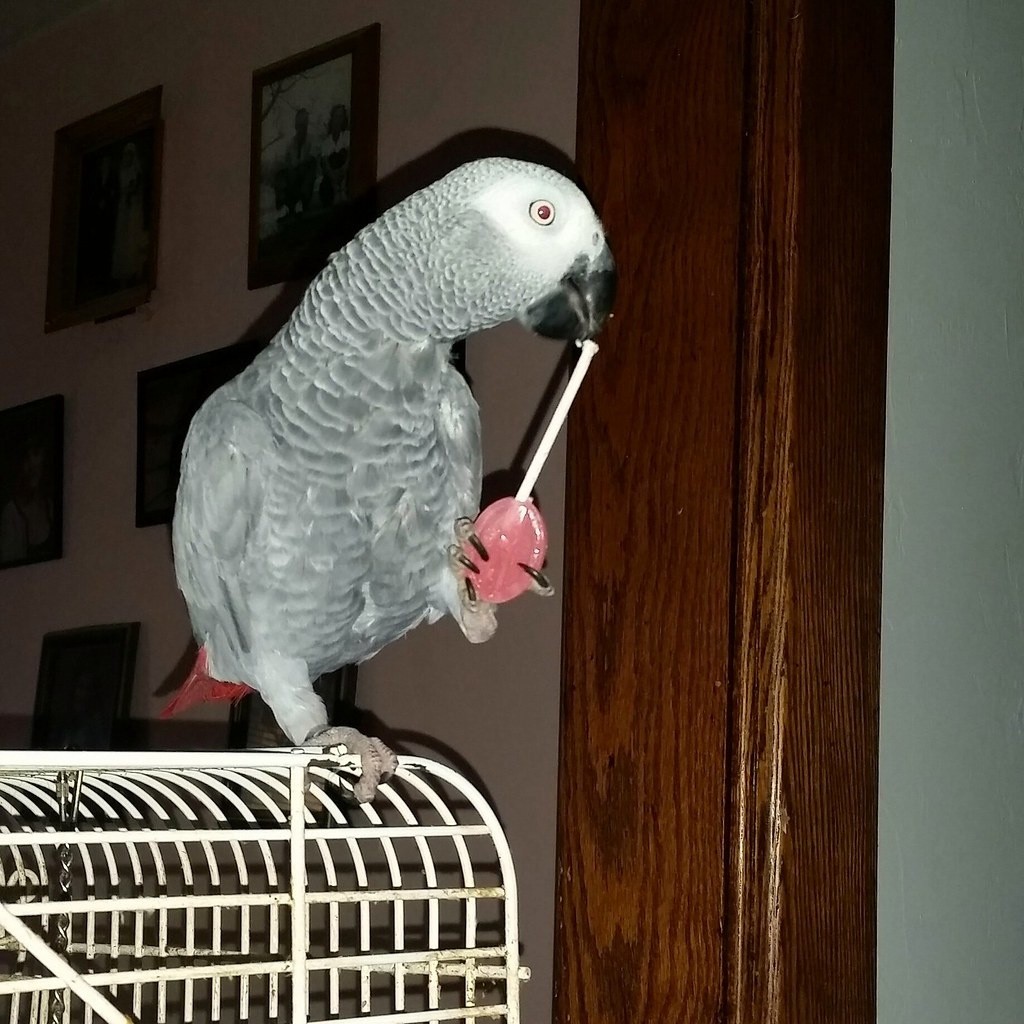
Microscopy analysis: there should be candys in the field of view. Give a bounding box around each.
[463,339,599,603]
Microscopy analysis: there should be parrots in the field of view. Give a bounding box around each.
[160,157,615,803]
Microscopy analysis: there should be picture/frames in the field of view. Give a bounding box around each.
[222,664,358,832]
[136,339,256,528]
[0,392,66,571]
[247,19,383,292]
[34,621,141,750]
[40,84,162,335]
